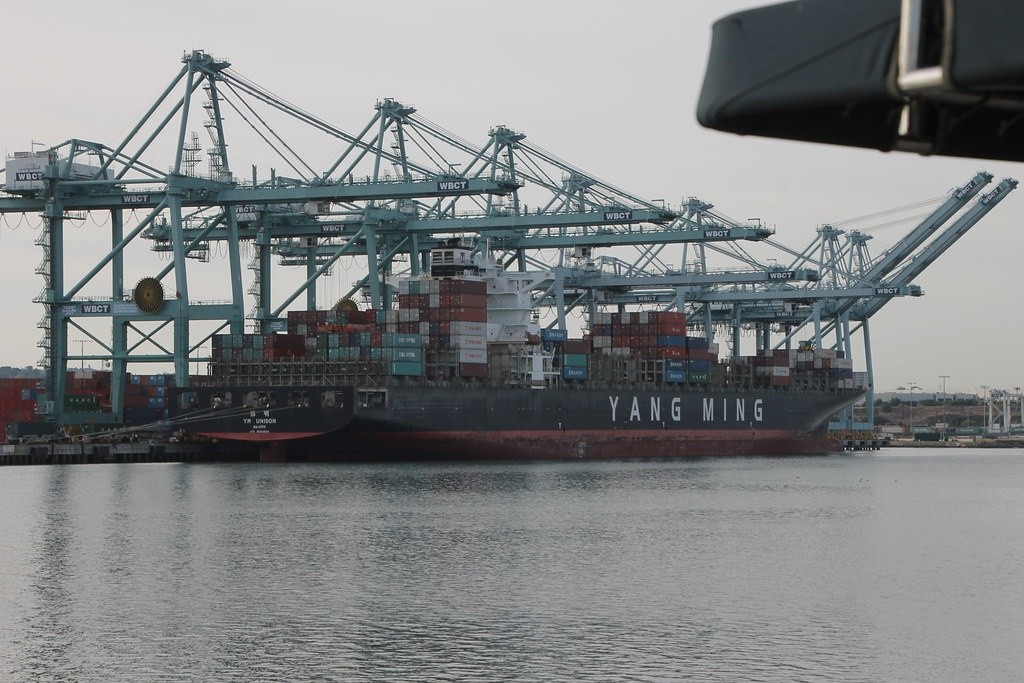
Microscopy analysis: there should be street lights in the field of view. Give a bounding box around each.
[938,375,951,440]
[907,381,916,431]
[980,385,990,428]
[73,339,93,396]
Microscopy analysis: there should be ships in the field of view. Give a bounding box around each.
[1,52,1023,456]
